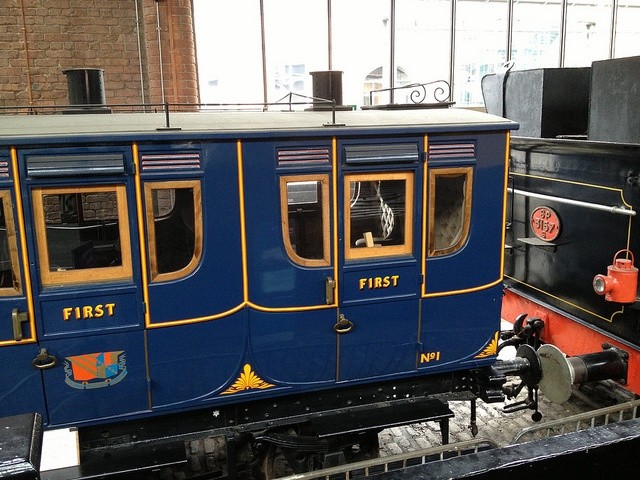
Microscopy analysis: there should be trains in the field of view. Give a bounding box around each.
[0,55,640,480]
